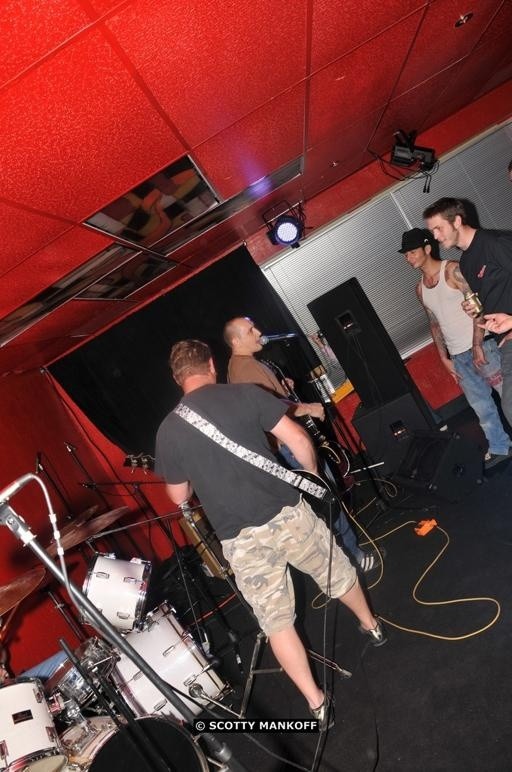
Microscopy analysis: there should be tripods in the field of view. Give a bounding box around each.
[68,456,151,561]
[78,478,264,665]
[90,503,260,668]
[90,502,353,721]
[40,469,102,558]
[35,473,250,772]
[286,340,428,539]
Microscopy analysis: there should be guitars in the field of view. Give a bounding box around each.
[258,355,355,490]
[122,444,344,535]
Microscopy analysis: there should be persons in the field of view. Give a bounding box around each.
[399,229,512,468]
[423,197,512,428]
[155,340,387,731]
[227,317,386,573]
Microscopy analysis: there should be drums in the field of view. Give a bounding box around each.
[78,550,158,633]
[44,634,123,709]
[1,674,68,772]
[59,716,209,772]
[108,598,235,729]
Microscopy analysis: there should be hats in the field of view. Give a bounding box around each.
[397,228,437,253]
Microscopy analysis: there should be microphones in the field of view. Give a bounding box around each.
[34,453,40,474]
[0,471,34,507]
[64,441,76,451]
[258,332,296,346]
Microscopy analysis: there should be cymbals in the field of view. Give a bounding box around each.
[42,503,132,560]
[48,502,106,542]
[1,566,47,618]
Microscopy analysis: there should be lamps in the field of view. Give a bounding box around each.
[261,199,315,251]
[368,129,439,193]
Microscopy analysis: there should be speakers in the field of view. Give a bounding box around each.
[350,390,431,476]
[306,277,438,444]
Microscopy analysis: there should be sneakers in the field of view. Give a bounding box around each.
[354,547,388,573]
[355,620,392,649]
[482,448,512,472]
[308,691,337,732]
